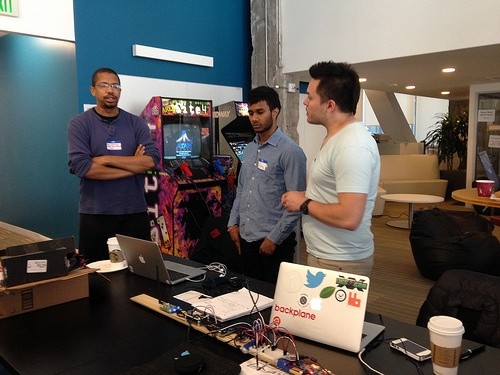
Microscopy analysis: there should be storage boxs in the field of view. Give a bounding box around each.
[0,235,100,319]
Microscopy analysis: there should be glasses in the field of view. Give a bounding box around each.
[95,82,122,91]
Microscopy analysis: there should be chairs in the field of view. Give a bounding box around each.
[416,269,500,351]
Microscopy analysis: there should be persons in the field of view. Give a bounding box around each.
[67,68,161,265]
[281,61,380,281]
[227,86,306,285]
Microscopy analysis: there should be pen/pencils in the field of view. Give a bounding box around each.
[460,344,485,363]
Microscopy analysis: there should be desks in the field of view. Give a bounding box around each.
[0,252,500,375]
[451,187,500,212]
[380,193,444,229]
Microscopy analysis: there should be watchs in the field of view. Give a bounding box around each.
[300,198,313,216]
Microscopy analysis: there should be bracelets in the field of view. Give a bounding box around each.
[227,224,238,232]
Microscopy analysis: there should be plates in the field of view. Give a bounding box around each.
[87,259,128,274]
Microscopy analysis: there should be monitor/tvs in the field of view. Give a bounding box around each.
[163,123,202,159]
[230,142,249,164]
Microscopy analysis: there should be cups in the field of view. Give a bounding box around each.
[476,180,495,198]
[428,315,465,375]
[107,237,125,263]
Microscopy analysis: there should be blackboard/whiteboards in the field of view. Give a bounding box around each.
[478,150,500,192]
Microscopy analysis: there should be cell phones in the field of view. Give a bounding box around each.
[390,338,431,362]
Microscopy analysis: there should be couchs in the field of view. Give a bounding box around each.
[371,154,449,216]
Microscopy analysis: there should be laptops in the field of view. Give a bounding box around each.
[477,151,500,191]
[269,261,385,352]
[116,234,207,285]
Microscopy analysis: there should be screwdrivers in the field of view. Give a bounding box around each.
[73,253,112,283]
